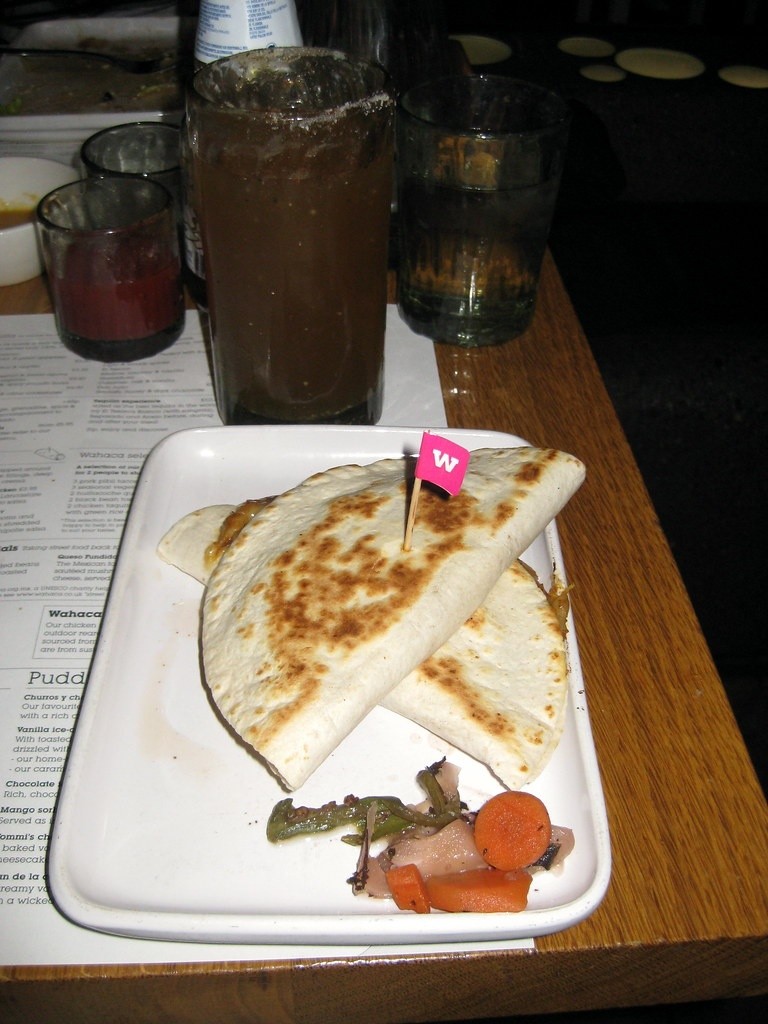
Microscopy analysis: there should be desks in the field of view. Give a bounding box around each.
[0,109,768,1023]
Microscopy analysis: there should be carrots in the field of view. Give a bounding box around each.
[386,790,551,913]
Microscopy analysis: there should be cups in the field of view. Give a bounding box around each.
[397,75,571,349]
[185,0,397,422]
[80,122,199,284]
[37,175,185,363]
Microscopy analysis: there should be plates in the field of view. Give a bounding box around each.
[44,426,611,945]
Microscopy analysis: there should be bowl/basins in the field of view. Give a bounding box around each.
[0,17,187,166]
[0,157,81,288]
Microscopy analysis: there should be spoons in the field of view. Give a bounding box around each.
[0,44,163,74]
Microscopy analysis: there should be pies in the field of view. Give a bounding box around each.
[158,493,568,793]
[199,445,586,789]
[443,33,768,88]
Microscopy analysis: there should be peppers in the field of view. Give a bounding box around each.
[267,764,463,844]
